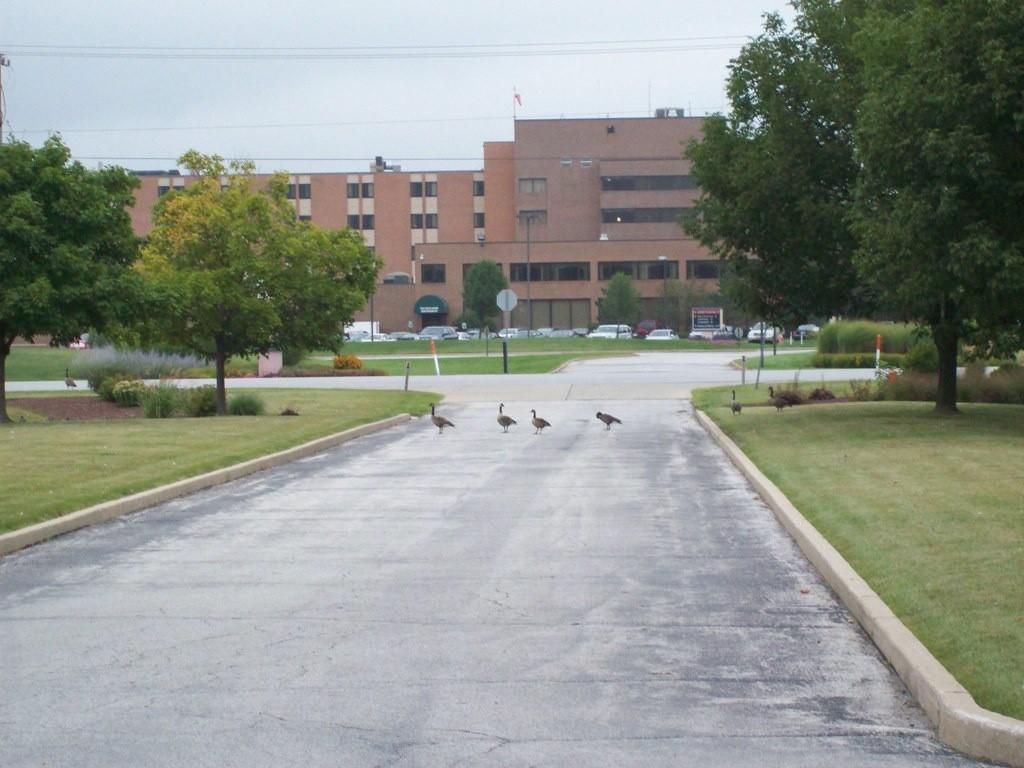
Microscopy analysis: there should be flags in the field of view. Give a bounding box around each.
[513,87,522,106]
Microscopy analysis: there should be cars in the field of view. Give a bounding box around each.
[748,321,780,344]
[712,325,737,340]
[456,327,589,340]
[792,324,820,341]
[688,330,717,340]
[645,329,679,340]
[69,334,91,351]
[343,330,419,342]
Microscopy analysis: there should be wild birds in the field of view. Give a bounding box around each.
[496,403,517,433]
[731,390,741,416]
[596,412,622,429]
[429,403,455,433]
[530,409,551,434]
[768,386,789,410]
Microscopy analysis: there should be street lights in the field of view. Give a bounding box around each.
[514,213,543,329]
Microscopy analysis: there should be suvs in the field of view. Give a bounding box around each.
[414,325,458,342]
[636,319,665,338]
[587,324,633,340]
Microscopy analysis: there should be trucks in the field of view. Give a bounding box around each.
[343,320,380,334]
